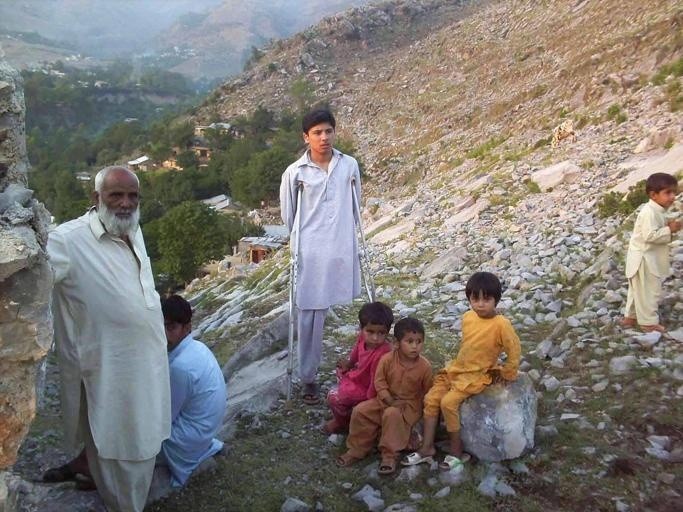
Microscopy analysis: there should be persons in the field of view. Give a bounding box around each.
[336,317,433,474]
[43,295,226,492]
[400,272,521,470]
[619,172,683,332]
[279,109,362,406]
[323,301,394,436]
[46,165,172,512]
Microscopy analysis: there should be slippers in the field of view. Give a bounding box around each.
[319,420,350,436]
[439,452,471,469]
[301,384,320,405]
[377,455,398,475]
[640,324,665,333]
[42,462,85,482]
[400,452,434,466]
[74,473,96,491]
[335,449,362,467]
[619,317,637,327]
